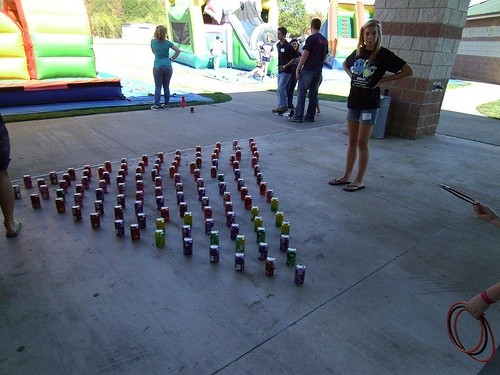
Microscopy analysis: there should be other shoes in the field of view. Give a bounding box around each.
[151,105,159,111]
[162,104,170,109]
[288,117,302,122]
[272,108,285,113]
[303,116,314,122]
[288,105,294,108]
[317,110,320,113]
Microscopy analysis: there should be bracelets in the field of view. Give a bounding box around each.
[481,289,496,304]
[171,57,173,60]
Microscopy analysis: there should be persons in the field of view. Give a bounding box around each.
[260,36,273,81]
[272,27,297,114]
[0,113,22,237]
[210,36,223,71]
[466,202,500,375]
[151,25,180,110]
[287,39,302,108]
[288,19,328,123]
[329,19,413,191]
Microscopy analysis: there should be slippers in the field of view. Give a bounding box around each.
[329,178,351,185]
[6,219,22,238]
[343,183,365,192]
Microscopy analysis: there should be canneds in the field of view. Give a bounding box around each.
[59,180,68,194]
[83,170,91,182]
[23,175,33,189]
[62,173,72,185]
[74,193,84,209]
[40,185,50,200]
[89,137,306,286]
[76,183,85,198]
[12,185,22,199]
[84,165,92,176]
[71,205,82,222]
[56,188,66,202]
[37,179,48,189]
[191,107,194,113]
[29,192,41,209]
[49,171,58,185]
[81,176,90,190]
[67,168,76,181]
[55,198,66,214]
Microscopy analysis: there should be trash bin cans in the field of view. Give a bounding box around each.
[369,95,392,139]
